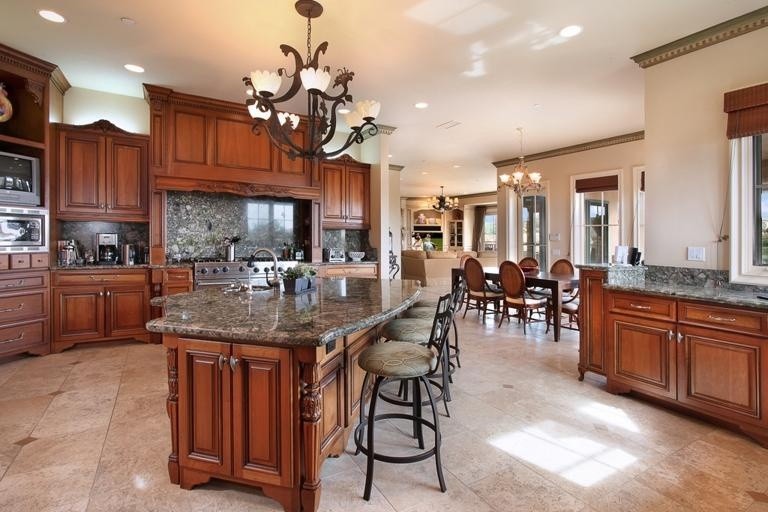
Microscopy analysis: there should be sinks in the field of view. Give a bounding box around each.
[222,285,272,292]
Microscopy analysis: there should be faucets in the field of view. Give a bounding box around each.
[247,248,281,287]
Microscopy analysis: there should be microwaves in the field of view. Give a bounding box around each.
[0,215,42,246]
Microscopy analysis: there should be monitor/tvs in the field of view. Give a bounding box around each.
[0,151,40,206]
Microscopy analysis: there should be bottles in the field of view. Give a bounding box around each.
[86,255,95,266]
[282,242,305,260]
[61,242,75,266]
[417,213,425,224]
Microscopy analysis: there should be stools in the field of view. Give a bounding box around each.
[382,294,451,439]
[355,309,454,500]
[409,273,466,402]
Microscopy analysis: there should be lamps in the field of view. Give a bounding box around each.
[497,127,546,200]
[241,0,382,163]
[427,186,459,215]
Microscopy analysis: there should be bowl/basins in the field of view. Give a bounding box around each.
[348,251,365,262]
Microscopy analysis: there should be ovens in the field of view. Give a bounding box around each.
[194,279,240,291]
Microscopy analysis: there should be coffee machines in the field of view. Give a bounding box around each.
[96,232,119,264]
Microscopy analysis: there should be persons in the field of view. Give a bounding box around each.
[411,232,436,251]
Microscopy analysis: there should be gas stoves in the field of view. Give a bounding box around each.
[188,253,295,276]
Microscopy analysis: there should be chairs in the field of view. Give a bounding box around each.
[452,253,581,341]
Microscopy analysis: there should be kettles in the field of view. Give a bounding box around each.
[120,242,137,266]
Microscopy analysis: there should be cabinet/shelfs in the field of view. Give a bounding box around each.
[51,269,152,354]
[56,119,150,224]
[602,290,768,449]
[319,154,370,232]
[0,252,50,362]
[160,335,317,512]
